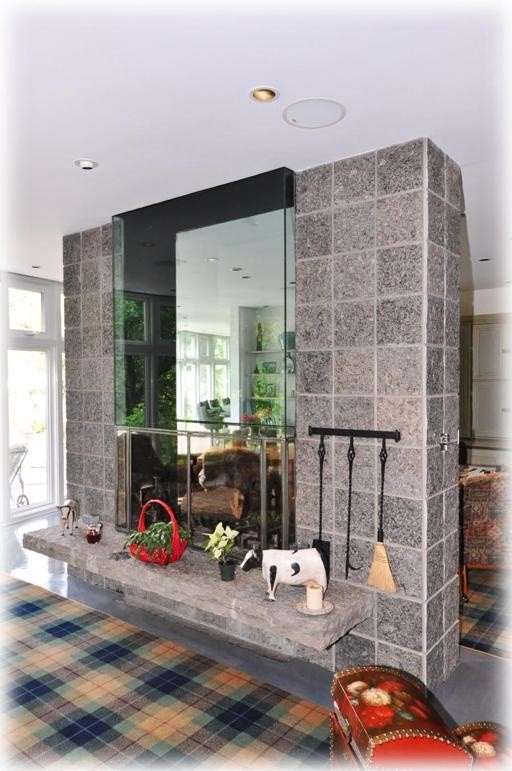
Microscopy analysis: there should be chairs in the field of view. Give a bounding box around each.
[196,397,231,446]
[459,471,512,596]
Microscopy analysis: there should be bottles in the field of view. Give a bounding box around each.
[255,322,264,351]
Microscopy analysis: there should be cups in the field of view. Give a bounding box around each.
[307,585,324,611]
[87,523,101,544]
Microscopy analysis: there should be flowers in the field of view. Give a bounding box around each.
[241,414,266,430]
[204,521,242,562]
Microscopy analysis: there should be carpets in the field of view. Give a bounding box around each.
[1,571,332,771]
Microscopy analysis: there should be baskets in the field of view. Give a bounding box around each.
[129,499,186,566]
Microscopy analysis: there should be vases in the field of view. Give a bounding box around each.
[218,561,236,583]
[246,429,260,450]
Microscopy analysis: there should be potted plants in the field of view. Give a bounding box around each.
[123,500,192,567]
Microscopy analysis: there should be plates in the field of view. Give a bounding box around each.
[297,600,335,616]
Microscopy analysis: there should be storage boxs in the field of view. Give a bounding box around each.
[329,665,512,771]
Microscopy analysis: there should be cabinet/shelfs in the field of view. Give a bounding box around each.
[245,350,295,425]
[472,324,512,439]
